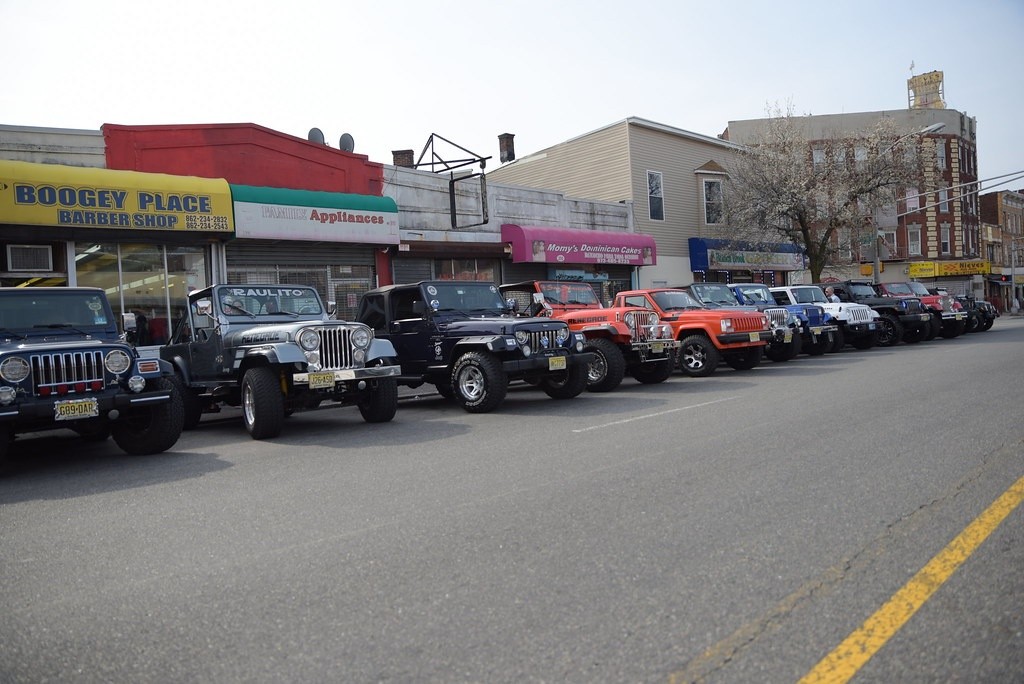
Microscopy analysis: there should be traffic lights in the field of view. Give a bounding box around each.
[880,262,885,273]
[1001,274,1007,281]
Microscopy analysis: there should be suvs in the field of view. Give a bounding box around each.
[726,282,838,362]
[355,279,594,413]
[820,280,931,347]
[768,285,880,353]
[611,289,772,377]
[928,288,1000,334]
[872,281,968,340]
[158,284,403,441]
[496,279,681,392]
[0,287,185,458]
[683,281,802,362]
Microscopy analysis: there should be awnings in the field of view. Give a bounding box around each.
[230,183,400,245]
[910,260,990,278]
[688,237,805,271]
[0,159,235,232]
[501,224,656,266]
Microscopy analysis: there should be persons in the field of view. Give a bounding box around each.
[965,290,972,299]
[825,286,840,303]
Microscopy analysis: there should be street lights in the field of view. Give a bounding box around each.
[874,122,947,162]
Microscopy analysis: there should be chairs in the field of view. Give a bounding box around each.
[197,327,215,343]
